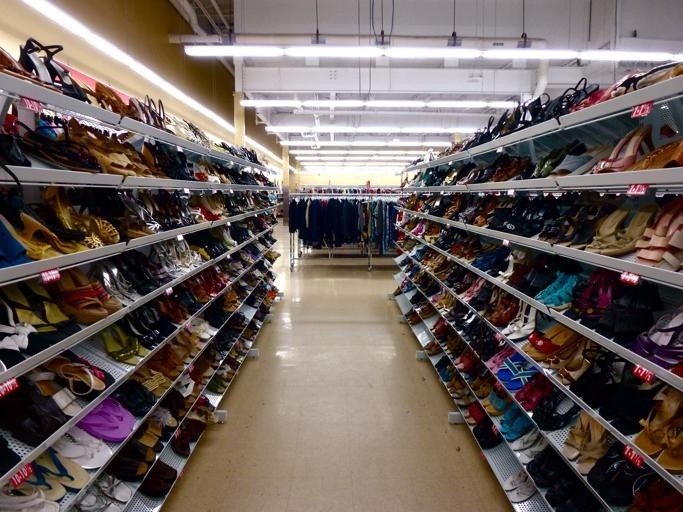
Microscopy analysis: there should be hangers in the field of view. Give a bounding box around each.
[291,192,410,203]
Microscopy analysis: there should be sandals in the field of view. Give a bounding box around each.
[0,38,282,508]
[392,57,683,510]
